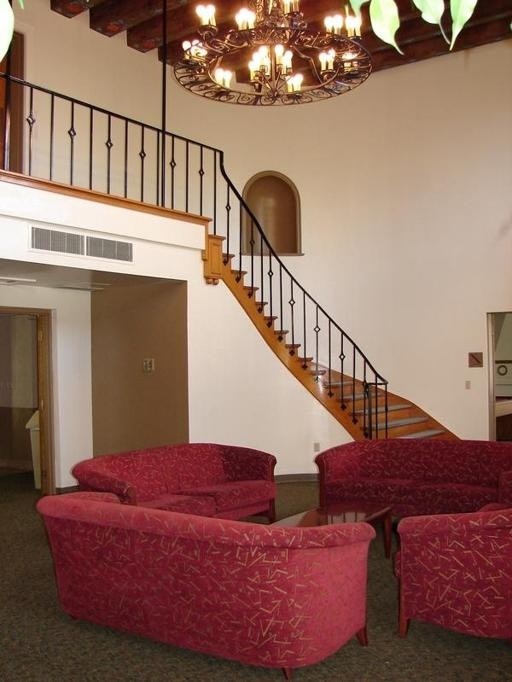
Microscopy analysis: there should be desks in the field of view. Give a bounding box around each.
[269,494,394,561]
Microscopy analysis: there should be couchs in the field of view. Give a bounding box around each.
[314,436,512,518]
[71,441,278,523]
[34,491,380,682]
[393,502,512,639]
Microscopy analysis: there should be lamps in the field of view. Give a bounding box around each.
[174,0,373,107]
